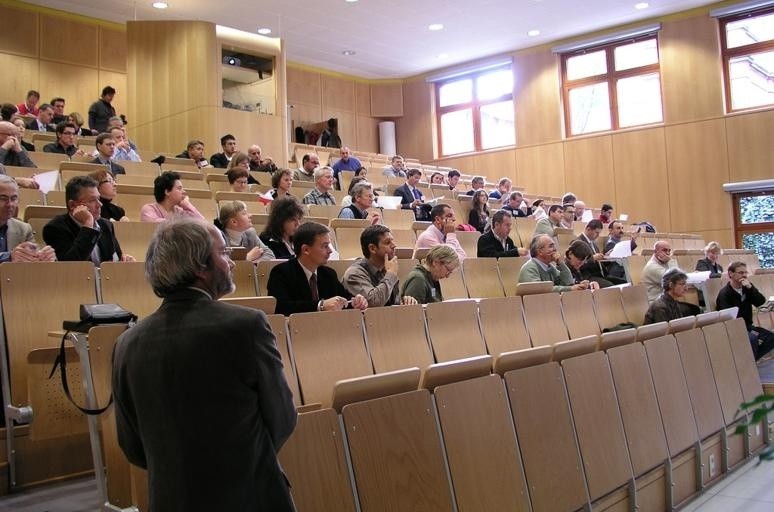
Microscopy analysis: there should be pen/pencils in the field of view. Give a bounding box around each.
[576,280,579,284]
[347,300,353,304]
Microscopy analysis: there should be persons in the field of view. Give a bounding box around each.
[109,219,297,512]
[6,85,774,363]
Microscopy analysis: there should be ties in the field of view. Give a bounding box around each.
[308,272,319,303]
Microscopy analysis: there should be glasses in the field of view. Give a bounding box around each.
[61,130,76,135]
[673,282,688,286]
[102,177,119,183]
[206,246,233,259]
[478,194,488,198]
[734,270,749,276]
[308,161,320,165]
[441,263,453,275]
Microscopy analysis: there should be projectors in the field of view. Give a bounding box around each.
[223,56,241,66]
